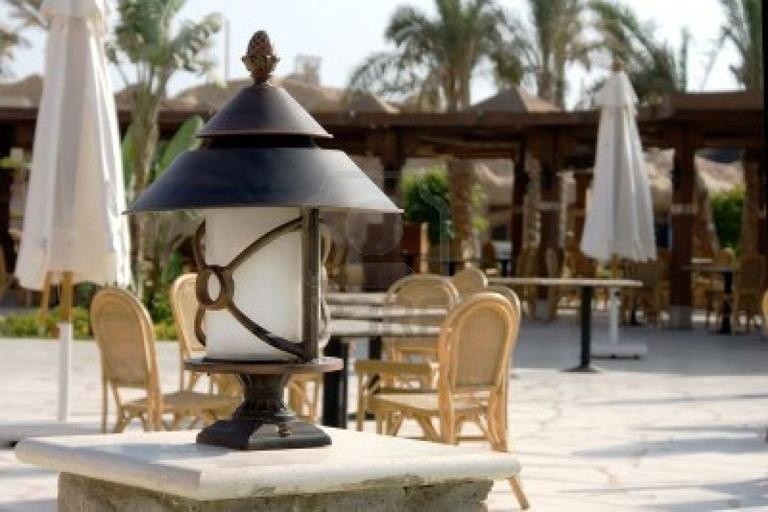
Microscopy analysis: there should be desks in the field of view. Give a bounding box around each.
[445,273,644,373]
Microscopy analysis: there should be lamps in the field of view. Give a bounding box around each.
[122,31,409,450]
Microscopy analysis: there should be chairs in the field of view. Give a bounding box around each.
[474,238,767,345]
[169,273,241,428]
[368,293,531,510]
[284,265,521,445]
[89,287,233,433]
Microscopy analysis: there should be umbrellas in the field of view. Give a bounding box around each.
[14,0,131,423]
[578,64,659,346]
